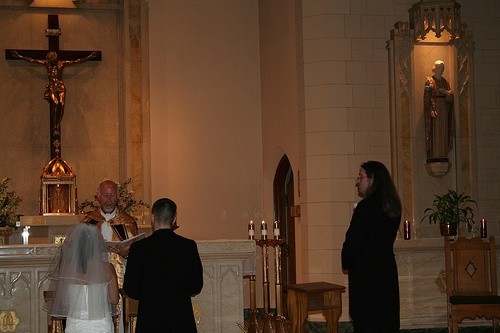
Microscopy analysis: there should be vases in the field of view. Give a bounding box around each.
[0,227,15,245]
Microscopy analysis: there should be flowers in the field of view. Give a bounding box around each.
[0,177,24,229]
[77,177,151,214]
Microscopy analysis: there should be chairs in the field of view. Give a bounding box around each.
[44,289,138,333]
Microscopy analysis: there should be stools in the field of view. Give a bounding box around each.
[284,282,346,333]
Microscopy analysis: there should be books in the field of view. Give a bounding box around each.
[104,232,145,251]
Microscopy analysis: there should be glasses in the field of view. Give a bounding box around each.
[356,176,364,183]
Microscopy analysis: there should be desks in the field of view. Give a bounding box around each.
[0,240,257,333]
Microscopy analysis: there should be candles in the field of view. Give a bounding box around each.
[248,220,255,240]
[404,220,411,240]
[273,221,280,240]
[480,218,488,238]
[261,221,267,241]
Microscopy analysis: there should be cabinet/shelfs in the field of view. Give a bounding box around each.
[445,236,500,333]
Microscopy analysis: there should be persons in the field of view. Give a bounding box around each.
[423,60,455,159]
[10,50,97,136]
[43,225,119,333]
[341,161,402,333]
[123,198,203,333]
[80,181,138,333]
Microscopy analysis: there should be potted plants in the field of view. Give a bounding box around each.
[420,188,478,236]
